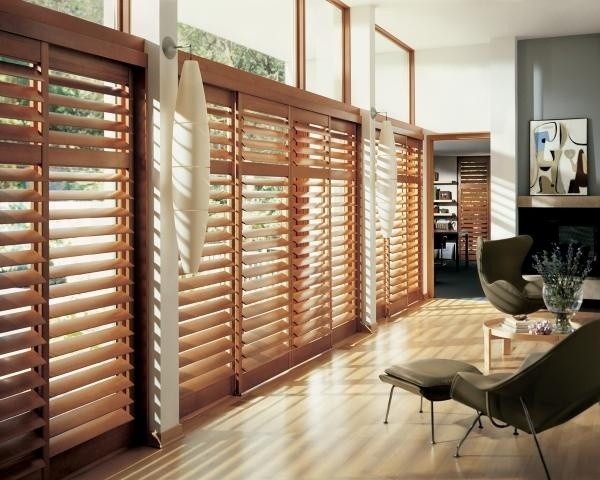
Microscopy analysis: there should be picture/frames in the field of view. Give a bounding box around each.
[528,117,589,196]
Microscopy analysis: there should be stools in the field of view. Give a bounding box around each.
[380,355,482,449]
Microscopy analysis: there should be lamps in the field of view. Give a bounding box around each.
[163,39,211,274]
[372,110,397,243]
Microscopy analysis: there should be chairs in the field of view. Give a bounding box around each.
[451,234,600,479]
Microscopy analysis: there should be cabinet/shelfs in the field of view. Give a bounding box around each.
[433,181,458,233]
